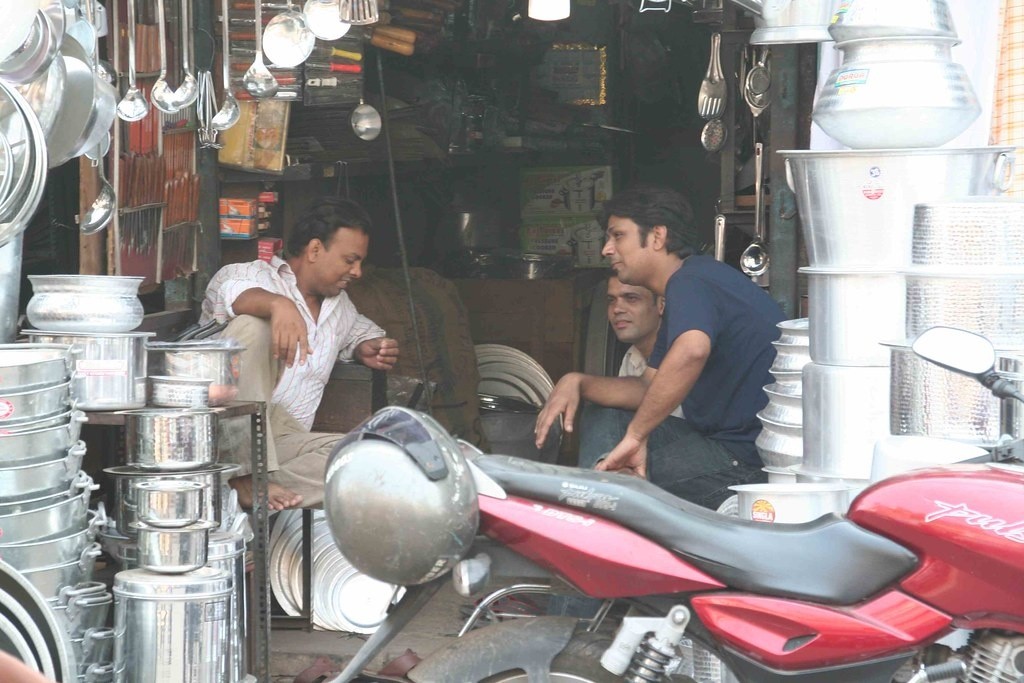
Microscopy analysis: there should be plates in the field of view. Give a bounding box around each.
[270,508,407,636]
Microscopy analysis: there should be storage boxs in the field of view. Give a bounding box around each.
[219,218,258,238]
[219,198,258,219]
[219,100,291,175]
[258,192,279,203]
[518,164,618,221]
[519,222,611,268]
[258,236,283,254]
[258,252,273,262]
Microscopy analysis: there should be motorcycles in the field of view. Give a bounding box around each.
[327,325,1024,682]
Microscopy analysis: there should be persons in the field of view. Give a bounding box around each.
[477,185,790,627]
[187,196,401,512]
[600,270,688,420]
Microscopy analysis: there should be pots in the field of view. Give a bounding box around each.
[568,223,606,261]
[559,171,603,211]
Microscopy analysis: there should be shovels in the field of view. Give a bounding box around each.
[339,0,381,26]
[696,31,728,122]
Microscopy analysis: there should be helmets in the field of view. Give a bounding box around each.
[321,406,479,587]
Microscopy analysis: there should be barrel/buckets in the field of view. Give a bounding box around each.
[0,275,251,683]
[727,0,1024,526]
[457,210,502,249]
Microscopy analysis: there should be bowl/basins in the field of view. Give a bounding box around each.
[520,254,549,280]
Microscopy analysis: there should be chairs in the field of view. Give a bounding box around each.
[310,363,388,433]
